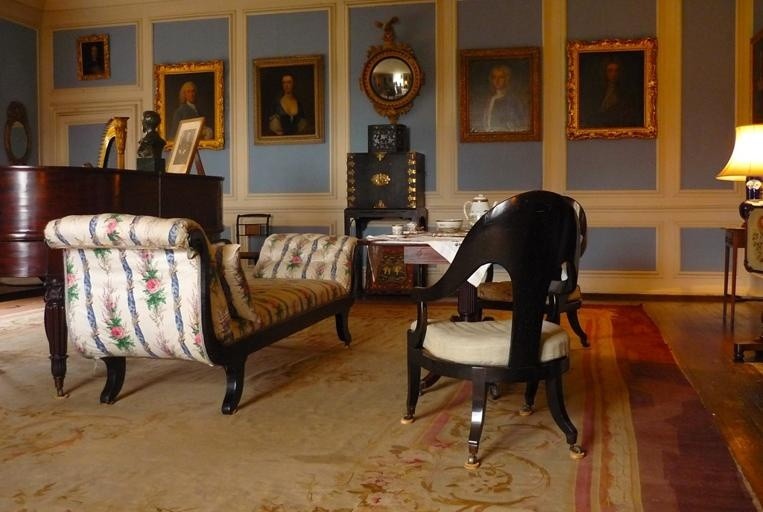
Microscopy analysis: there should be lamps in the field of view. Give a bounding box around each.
[713,122,762,227]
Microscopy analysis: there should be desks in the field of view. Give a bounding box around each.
[719,226,745,331]
[1,163,225,288]
[358,230,504,401]
[343,208,427,303]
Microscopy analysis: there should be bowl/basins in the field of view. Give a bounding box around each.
[436,219,462,234]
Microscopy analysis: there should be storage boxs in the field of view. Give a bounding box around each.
[346,152,426,210]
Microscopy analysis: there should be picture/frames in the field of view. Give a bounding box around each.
[251,53,327,145]
[153,58,225,152]
[165,115,207,175]
[564,36,658,140]
[76,32,111,81]
[455,45,542,143]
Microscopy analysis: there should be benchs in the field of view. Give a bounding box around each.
[41,212,361,415]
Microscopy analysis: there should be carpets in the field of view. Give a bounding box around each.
[0,300,761,510]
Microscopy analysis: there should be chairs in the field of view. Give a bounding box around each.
[734,208,762,360]
[398,187,586,472]
[236,213,271,266]
[475,194,591,349]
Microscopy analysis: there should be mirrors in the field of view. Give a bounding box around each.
[358,16,425,124]
[4,101,33,165]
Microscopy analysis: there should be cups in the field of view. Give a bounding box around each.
[392,224,403,235]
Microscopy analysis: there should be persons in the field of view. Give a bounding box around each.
[586,59,639,129]
[170,80,214,140]
[474,64,530,131]
[136,110,168,160]
[267,73,310,136]
[174,130,194,164]
[85,44,104,75]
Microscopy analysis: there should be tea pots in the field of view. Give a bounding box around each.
[463,193,497,227]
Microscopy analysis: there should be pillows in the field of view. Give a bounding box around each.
[210,240,263,326]
